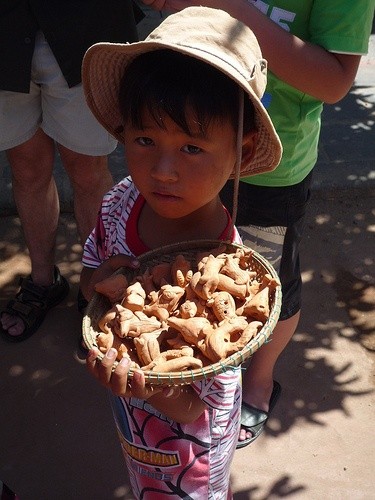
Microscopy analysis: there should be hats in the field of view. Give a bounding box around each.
[82,6,284,180]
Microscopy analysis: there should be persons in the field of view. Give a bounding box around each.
[76,5,284,500]
[0,0,139,353]
[138,0,375,449]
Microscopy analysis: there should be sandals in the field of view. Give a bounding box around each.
[1,265,70,342]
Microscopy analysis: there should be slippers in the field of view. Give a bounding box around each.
[236,382,282,446]
